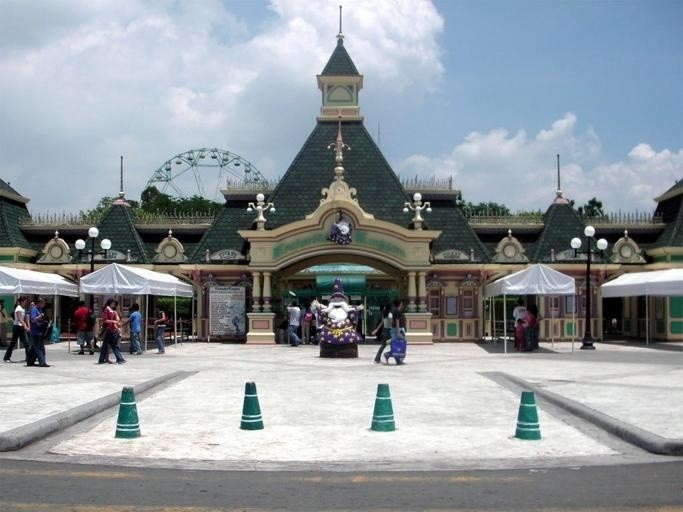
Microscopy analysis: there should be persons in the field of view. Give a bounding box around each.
[0,296,142,367]
[286,301,314,346]
[371,296,407,366]
[154,303,167,354]
[513,299,543,352]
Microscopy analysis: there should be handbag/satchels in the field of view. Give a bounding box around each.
[98,325,119,345]
[391,340,406,356]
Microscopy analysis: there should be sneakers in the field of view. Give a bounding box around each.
[79,350,84,354]
[90,348,95,354]
[27,361,38,366]
[39,362,49,367]
[3,359,11,362]
[99,357,125,364]
[376,352,406,365]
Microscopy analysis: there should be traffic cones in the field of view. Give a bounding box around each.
[368,382,397,432]
[510,388,544,441]
[109,384,143,438]
[236,381,266,430]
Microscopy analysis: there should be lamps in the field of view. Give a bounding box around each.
[403,193,432,230]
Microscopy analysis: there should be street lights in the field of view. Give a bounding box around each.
[72,226,113,311]
[568,224,612,351]
[401,191,434,230]
